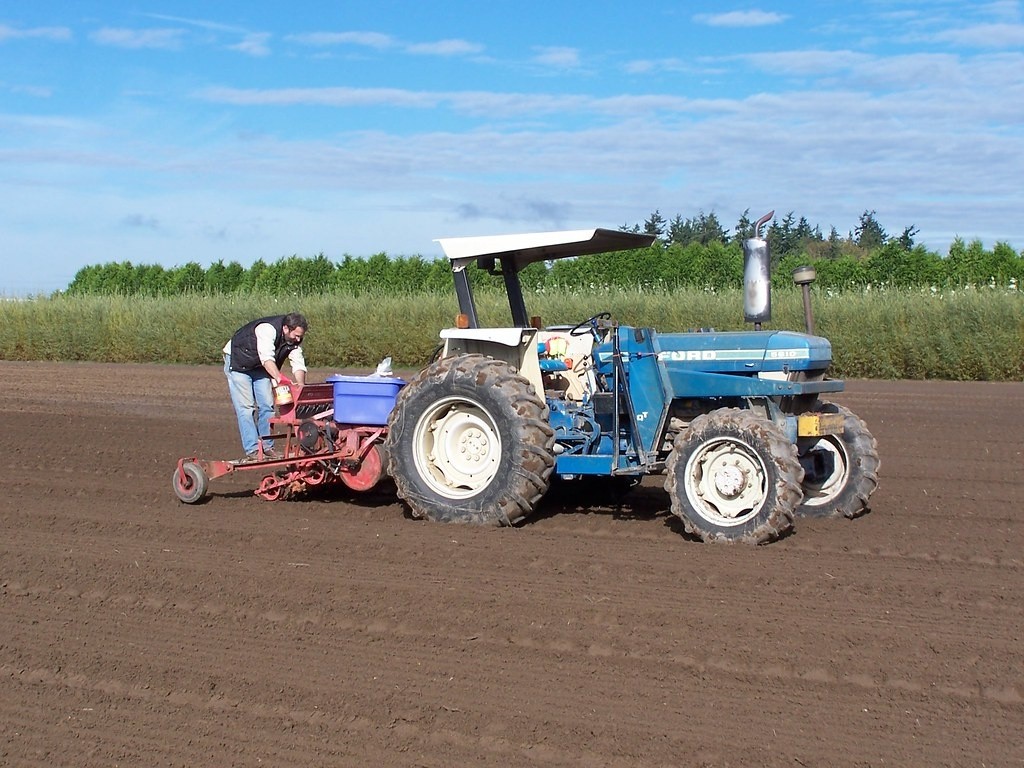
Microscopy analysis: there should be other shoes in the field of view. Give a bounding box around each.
[265,450,281,455]
[248,452,267,461]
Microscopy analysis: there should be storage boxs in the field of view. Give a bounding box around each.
[325,376,406,425]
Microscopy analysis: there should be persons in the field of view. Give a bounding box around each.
[221,312,308,458]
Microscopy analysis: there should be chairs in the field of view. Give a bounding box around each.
[537,342,568,372]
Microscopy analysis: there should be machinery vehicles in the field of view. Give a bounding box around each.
[381,208,882,546]
